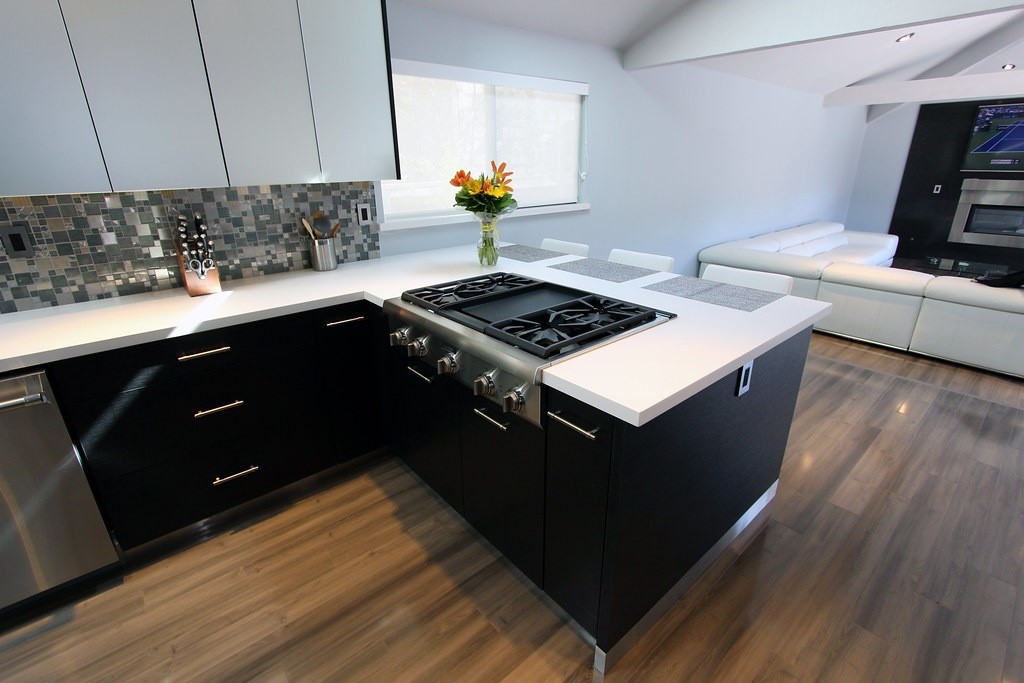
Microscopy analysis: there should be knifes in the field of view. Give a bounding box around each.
[173,210,216,270]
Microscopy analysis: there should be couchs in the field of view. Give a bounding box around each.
[697,221,1024,382]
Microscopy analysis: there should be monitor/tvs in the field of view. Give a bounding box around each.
[960,103,1024,172]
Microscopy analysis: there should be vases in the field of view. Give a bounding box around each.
[451,160,516,268]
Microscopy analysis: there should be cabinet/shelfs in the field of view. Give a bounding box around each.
[0,0,406,199]
[47,297,394,575]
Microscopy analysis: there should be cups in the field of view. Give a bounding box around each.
[308,236,339,271]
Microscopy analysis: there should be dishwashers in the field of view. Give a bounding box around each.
[0,370,125,629]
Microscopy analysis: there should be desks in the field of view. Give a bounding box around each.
[347,236,832,673]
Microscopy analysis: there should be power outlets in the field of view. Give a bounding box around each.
[357,204,373,230]
[932,184,942,194]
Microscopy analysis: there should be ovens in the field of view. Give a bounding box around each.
[947,178,1024,250]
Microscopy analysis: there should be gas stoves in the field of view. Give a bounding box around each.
[384,272,678,430]
